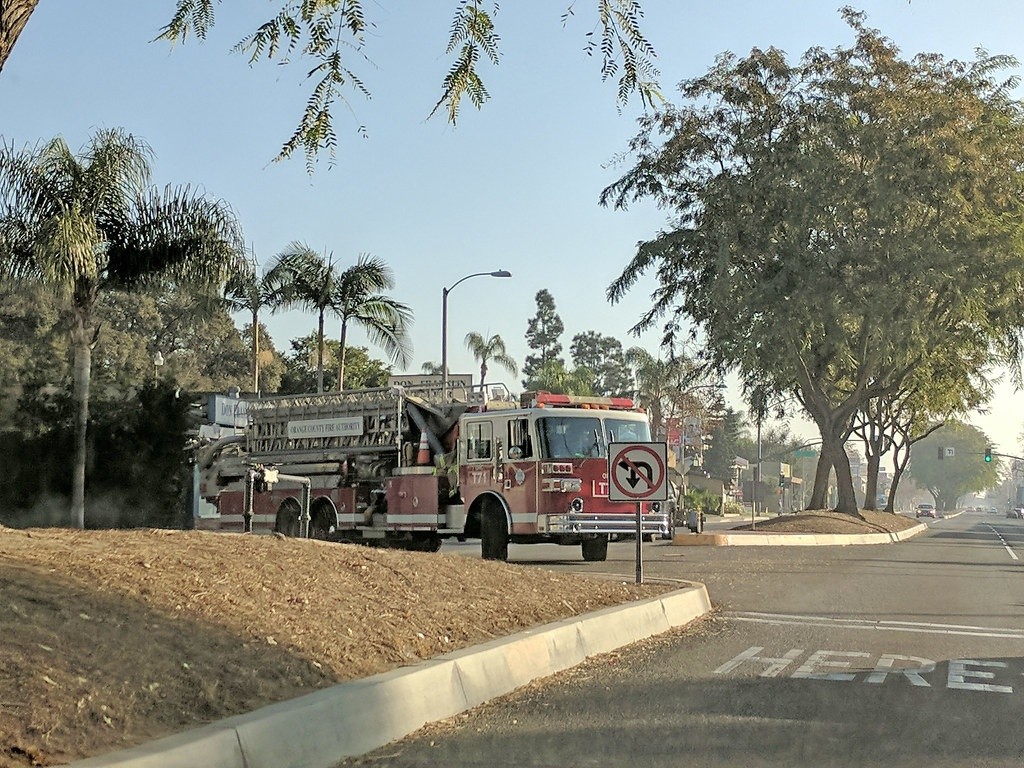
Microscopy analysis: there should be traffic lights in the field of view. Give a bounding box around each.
[984,448,992,462]
[778,474,785,486]
[938,447,943,459]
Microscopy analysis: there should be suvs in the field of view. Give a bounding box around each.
[1015,508,1024,518]
[617,480,676,541]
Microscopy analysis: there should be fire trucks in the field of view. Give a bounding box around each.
[194,380,667,563]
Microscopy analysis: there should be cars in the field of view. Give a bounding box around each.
[916,504,935,518]
[1006,510,1018,518]
[988,508,996,513]
[967,507,976,511]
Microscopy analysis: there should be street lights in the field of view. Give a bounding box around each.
[443,270,511,396]
[679,385,727,509]
